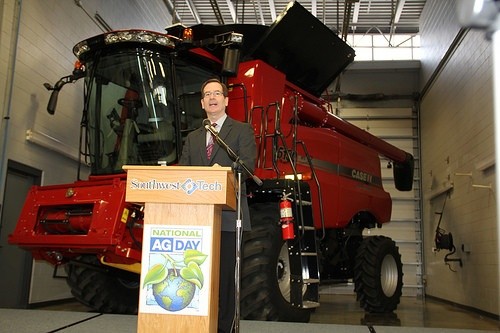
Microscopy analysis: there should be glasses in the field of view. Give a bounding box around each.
[204,92,224,97]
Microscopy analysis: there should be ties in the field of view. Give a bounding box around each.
[207,123,217,161]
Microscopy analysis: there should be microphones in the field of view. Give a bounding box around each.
[203,118,211,129]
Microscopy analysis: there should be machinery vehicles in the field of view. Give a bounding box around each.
[9,0,416,319]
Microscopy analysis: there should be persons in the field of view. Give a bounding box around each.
[177,78,258,333]
